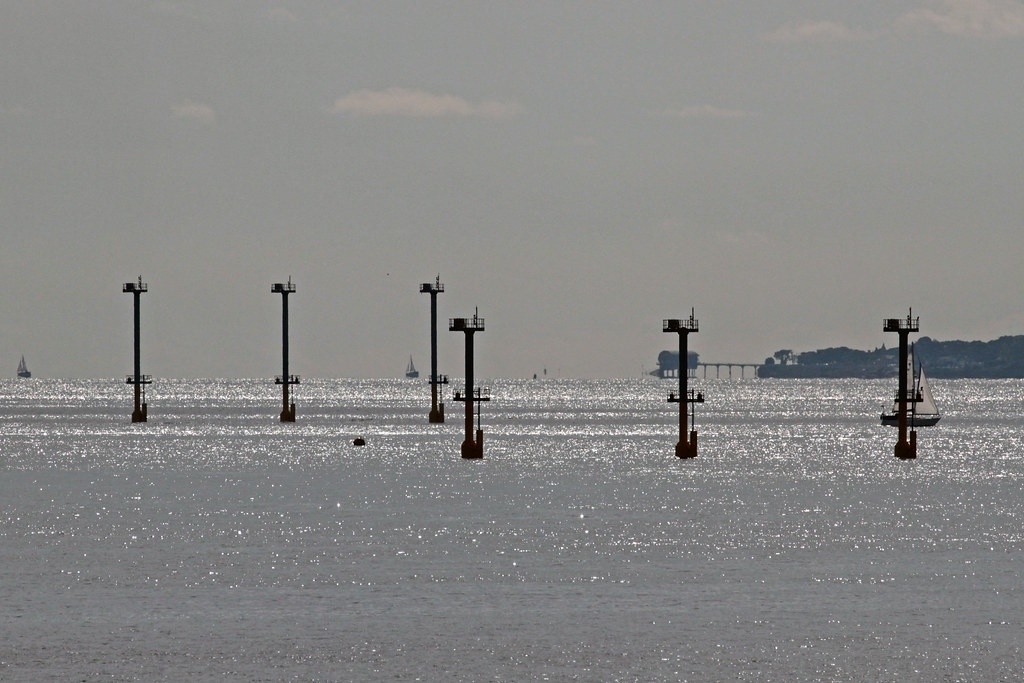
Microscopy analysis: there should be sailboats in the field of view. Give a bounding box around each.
[15,354,32,378]
[406,354,419,378]
[878,342,942,428]
